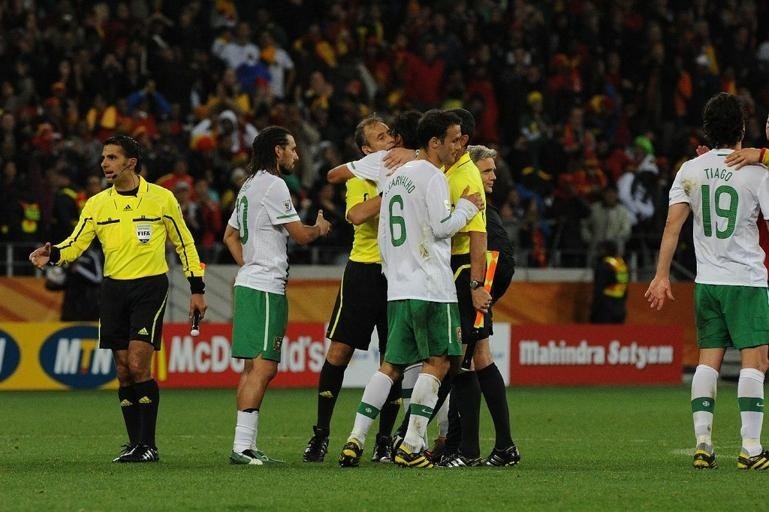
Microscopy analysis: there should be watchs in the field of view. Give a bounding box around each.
[468,280,485,289]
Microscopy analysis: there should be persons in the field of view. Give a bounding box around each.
[0,156,47,242]
[224,127,333,466]
[1,1,769,92]
[465,144,515,303]
[510,92,655,266]
[0,92,259,157]
[588,241,629,325]
[301,118,397,463]
[442,109,521,469]
[328,108,485,468]
[327,112,422,203]
[696,107,769,275]
[645,93,768,469]
[29,138,209,463]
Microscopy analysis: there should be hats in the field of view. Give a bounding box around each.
[599,238,618,252]
[633,133,655,153]
[232,168,245,179]
[527,91,544,104]
[442,98,462,110]
[176,182,189,192]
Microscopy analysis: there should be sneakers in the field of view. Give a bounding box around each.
[371,430,393,465]
[229,448,277,466]
[303,426,330,462]
[122,442,160,463]
[435,447,481,469]
[338,436,363,469]
[380,433,403,464]
[425,436,446,462]
[473,442,522,471]
[113,441,137,463]
[735,449,769,473]
[255,450,287,464]
[690,446,717,470]
[392,441,437,469]
